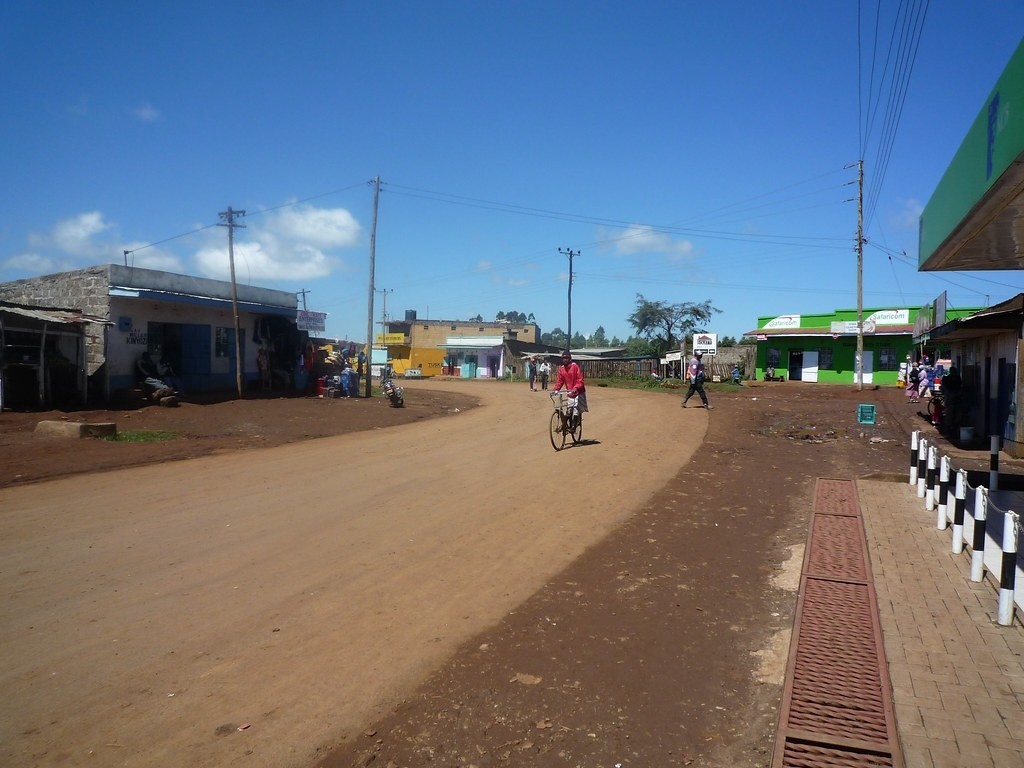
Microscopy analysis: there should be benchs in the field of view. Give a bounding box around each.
[763,376,784,382]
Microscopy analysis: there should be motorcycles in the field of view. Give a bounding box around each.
[378,376,405,408]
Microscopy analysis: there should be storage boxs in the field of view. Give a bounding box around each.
[323,375,343,399]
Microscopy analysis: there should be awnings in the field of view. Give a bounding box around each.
[436,343,502,349]
[742,323,914,337]
[0,306,117,325]
[912,306,1022,346]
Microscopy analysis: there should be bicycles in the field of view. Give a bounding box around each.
[549,390,582,452]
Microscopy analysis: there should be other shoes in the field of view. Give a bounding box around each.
[907,399,915,402]
[682,401,687,408]
[531,389,533,390]
[916,399,919,403]
[705,404,713,409]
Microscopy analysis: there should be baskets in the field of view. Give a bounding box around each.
[930,390,944,395]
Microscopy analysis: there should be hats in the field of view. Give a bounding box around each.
[913,362,918,367]
[919,365,924,369]
[531,359,535,362]
[695,350,702,355]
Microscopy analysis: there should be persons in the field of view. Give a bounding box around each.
[939,367,963,432]
[271,361,292,398]
[540,359,551,391]
[304,342,324,397]
[528,358,537,391]
[730,364,740,385]
[136,352,184,399]
[256,348,267,391]
[680,351,714,410]
[651,372,662,381]
[552,349,588,433]
[765,363,775,381]
[904,362,931,403]
[356,352,366,392]
[339,349,355,399]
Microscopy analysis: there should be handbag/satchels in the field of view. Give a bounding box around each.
[686,366,691,379]
